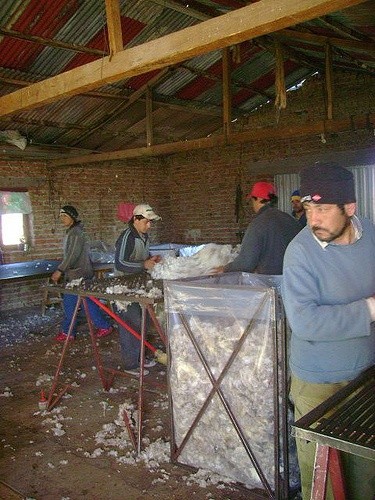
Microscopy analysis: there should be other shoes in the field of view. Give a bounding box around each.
[144,361,156,367]
[124,368,149,375]
[96,326,114,337]
[52,330,74,341]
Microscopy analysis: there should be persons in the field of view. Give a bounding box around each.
[113,204,162,376]
[208,181,303,275]
[281,161,375,500]
[51,206,114,342]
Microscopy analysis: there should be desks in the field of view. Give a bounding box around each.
[1,242,194,281]
[291,365,375,499]
[40,275,169,455]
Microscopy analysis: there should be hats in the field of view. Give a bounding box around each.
[250,182,274,199]
[290,190,300,202]
[298,161,356,204]
[61,206,78,218]
[133,204,162,221]
